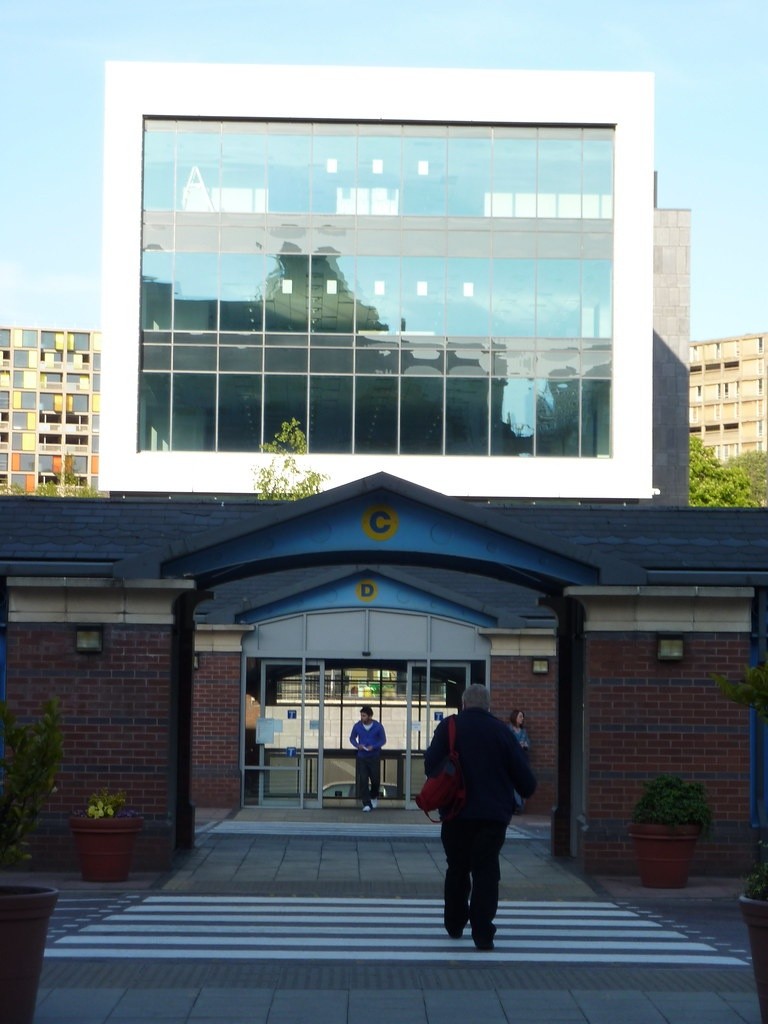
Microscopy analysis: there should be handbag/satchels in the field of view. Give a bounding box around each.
[415,717,466,824]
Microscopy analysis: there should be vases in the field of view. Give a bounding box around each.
[71,816,144,882]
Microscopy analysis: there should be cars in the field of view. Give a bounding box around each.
[358,685,374,692]
[319,781,405,808]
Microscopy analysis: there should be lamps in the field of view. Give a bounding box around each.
[658,635,685,661]
[76,623,105,653]
[533,658,548,676]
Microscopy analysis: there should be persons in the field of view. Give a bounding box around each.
[506,710,531,815]
[350,706,387,811]
[424,684,538,950]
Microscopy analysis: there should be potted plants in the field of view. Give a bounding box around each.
[629,774,712,888]
[1,696,70,1024]
[740,842,768,1023]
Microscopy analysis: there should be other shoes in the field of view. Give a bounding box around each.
[475,931,494,950]
[371,799,377,809]
[362,806,371,812]
[449,929,463,938]
[513,809,521,816]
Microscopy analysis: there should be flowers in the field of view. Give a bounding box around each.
[74,790,141,819]
